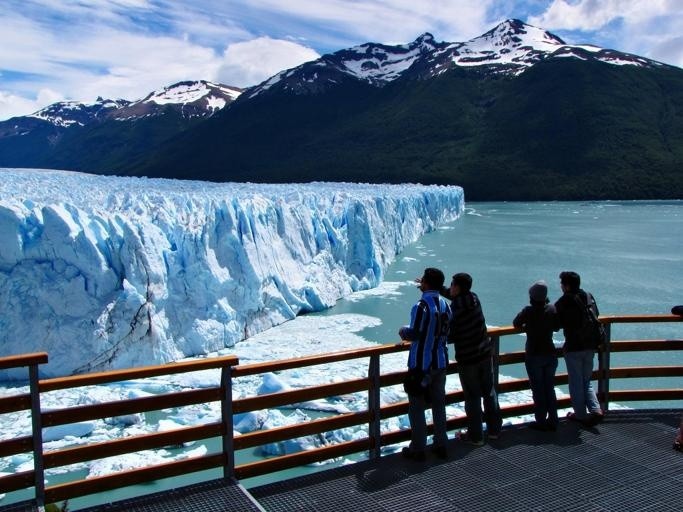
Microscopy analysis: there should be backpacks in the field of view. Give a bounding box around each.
[564,291,608,352]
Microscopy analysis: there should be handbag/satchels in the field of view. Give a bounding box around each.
[402,367,441,396]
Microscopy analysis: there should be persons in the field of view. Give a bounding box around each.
[398,267,454,458]
[553,271,605,425]
[669,305,682,452]
[414,273,502,449]
[512,279,561,432]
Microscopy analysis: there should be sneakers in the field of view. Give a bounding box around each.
[401,444,425,462]
[487,425,502,440]
[527,409,604,431]
[459,431,484,447]
[428,443,448,460]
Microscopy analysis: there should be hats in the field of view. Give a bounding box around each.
[528,283,548,302]
